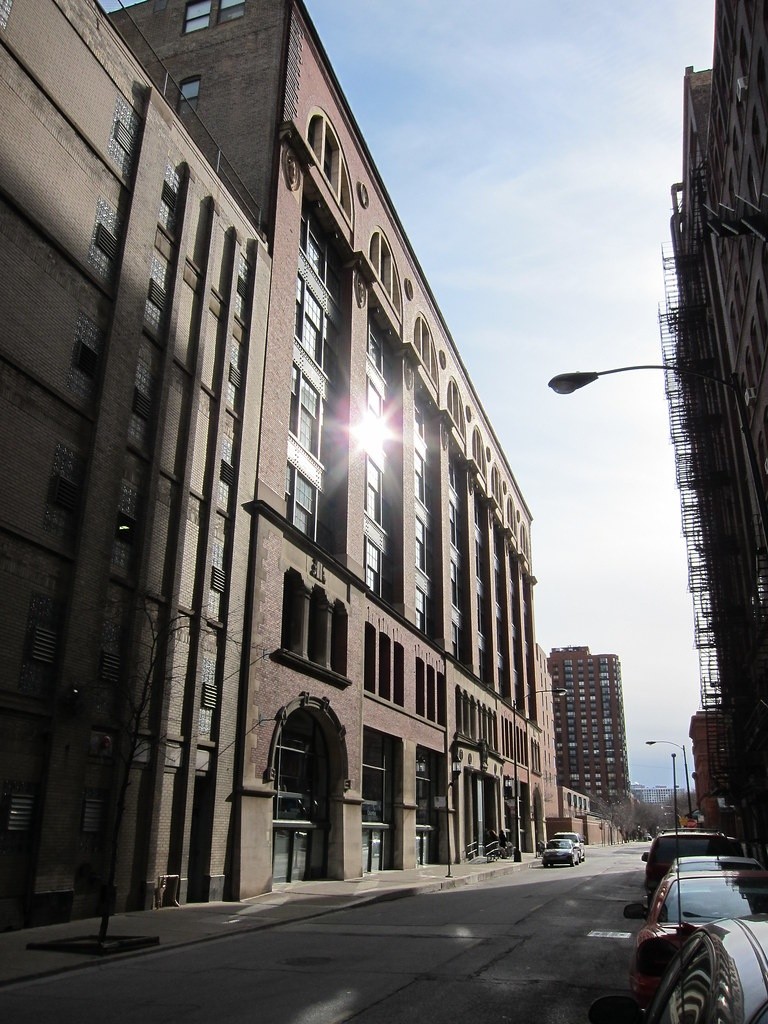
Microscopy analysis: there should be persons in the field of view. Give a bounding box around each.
[486,825,498,861]
[499,830,508,859]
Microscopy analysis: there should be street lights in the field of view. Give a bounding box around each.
[645,740,693,819]
[513,688,568,864]
[547,365,768,552]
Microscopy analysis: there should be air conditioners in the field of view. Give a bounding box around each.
[744,387,758,406]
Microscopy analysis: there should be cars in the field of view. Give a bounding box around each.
[665,855,768,901]
[622,869,768,1010]
[541,839,580,867]
[587,912,768,1024]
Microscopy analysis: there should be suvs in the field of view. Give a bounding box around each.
[641,828,745,908]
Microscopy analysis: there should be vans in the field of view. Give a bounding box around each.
[551,832,586,863]
